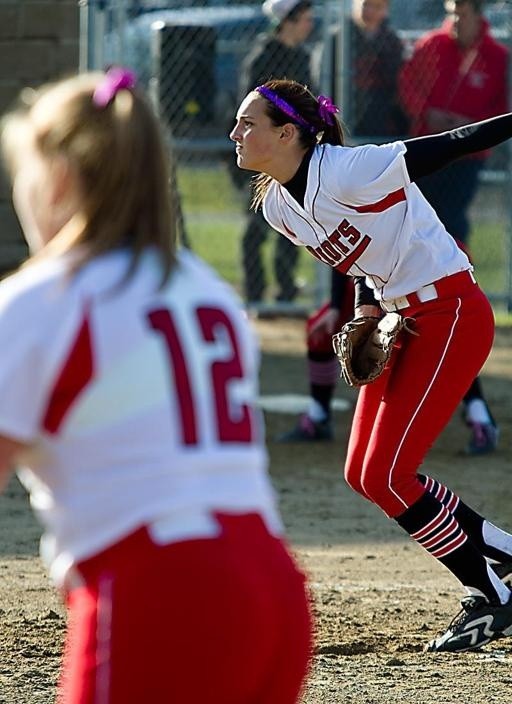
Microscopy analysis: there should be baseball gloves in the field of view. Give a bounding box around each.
[331,313,416,386]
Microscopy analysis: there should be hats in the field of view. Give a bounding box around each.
[262,0,297,28]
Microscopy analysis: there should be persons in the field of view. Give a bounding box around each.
[0,66,312,704]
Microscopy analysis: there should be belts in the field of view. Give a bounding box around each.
[379,271,478,313]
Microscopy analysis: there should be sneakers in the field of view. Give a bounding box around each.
[466,403,499,451]
[277,413,330,443]
[424,561,511,652]
